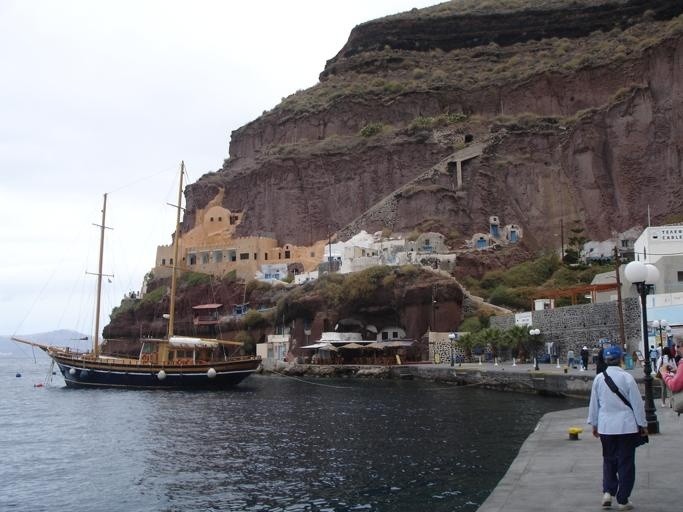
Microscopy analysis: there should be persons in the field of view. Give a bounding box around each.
[593,345,597,363]
[650,333,683,413]
[587,345,647,511]
[567,349,575,367]
[581,346,588,370]
[595,345,600,363]
[596,347,607,373]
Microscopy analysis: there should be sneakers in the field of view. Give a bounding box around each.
[618,501,634,511]
[602,492,612,506]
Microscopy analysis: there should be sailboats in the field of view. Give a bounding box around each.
[11,161,263,388]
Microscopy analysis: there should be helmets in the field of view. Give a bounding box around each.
[602,345,626,361]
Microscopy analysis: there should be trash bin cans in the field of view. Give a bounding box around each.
[549,355,558,364]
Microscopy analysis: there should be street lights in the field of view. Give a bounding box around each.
[624,261,671,433]
[529,329,541,370]
[448,333,455,366]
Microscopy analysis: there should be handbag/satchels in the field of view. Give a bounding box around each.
[671,391,683,413]
[635,425,648,447]
